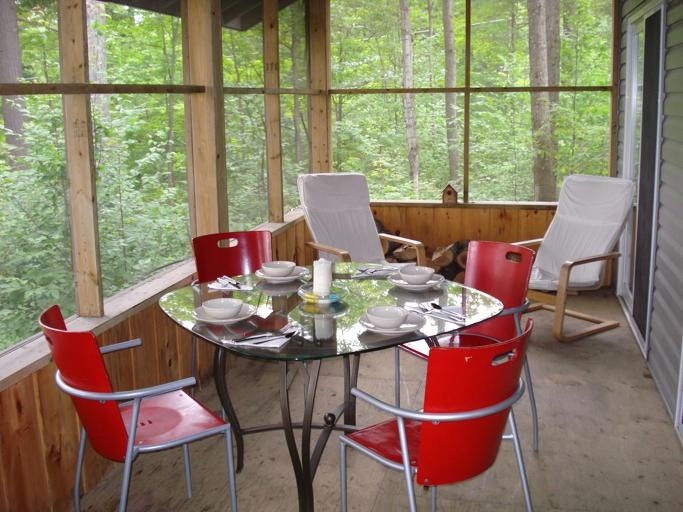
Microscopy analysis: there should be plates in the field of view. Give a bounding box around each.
[359,310,427,337]
[256,266,307,285]
[388,274,446,292]
[192,304,257,326]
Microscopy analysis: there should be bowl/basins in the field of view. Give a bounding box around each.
[261,261,297,278]
[399,266,435,285]
[366,307,409,331]
[202,298,244,320]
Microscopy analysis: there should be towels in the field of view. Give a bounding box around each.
[351,265,399,278]
[409,305,465,326]
[208,275,253,290]
[234,334,291,348]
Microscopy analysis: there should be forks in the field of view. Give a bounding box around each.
[419,304,465,328]
[233,325,290,342]
[364,270,398,275]
[222,278,240,289]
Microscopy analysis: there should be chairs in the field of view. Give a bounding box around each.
[393,240,538,453]
[510,175,634,342]
[190,230,287,401]
[336,316,533,511]
[37,304,237,512]
[296,172,425,267]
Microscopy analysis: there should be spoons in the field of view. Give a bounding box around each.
[431,302,466,320]
[222,273,241,285]
[356,267,382,273]
[254,332,296,345]
